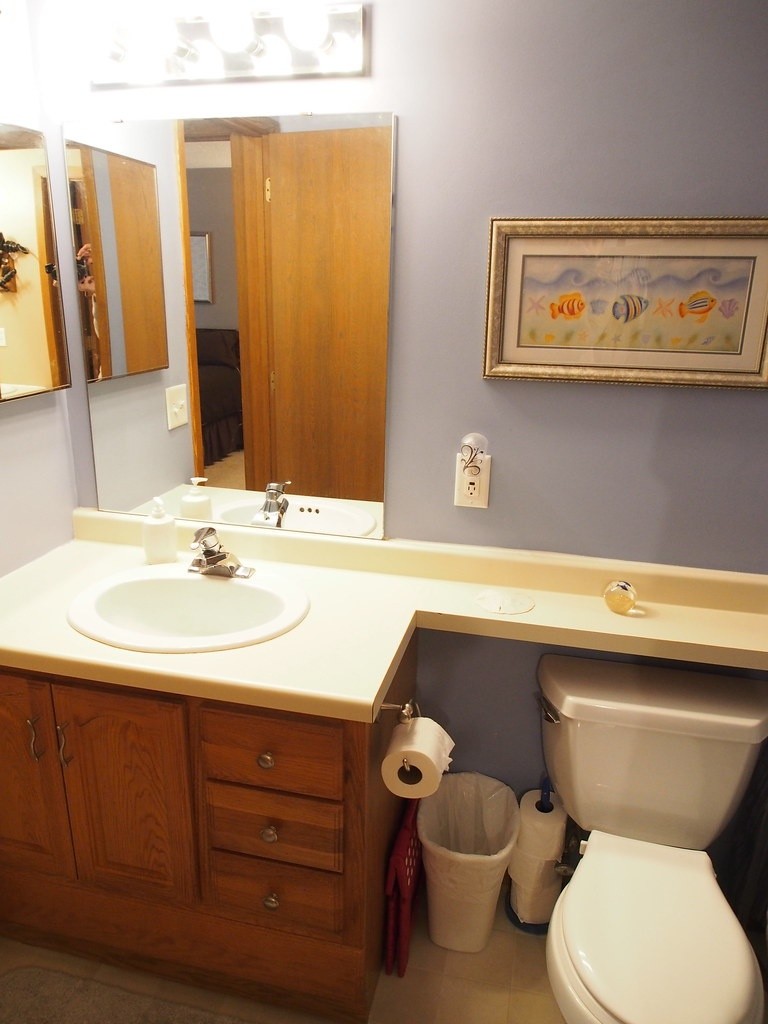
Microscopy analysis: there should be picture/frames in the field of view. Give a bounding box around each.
[189,231,212,303]
[481,218,768,392]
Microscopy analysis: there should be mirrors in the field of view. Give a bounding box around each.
[0,123,72,403]
[67,140,169,383]
[63,113,397,540]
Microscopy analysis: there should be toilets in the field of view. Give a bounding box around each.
[534,653,767,1022]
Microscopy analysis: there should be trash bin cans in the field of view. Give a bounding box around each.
[416,771,522,954]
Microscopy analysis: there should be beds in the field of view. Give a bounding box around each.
[197,327,243,465]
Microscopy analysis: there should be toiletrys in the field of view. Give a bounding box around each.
[180,476,210,521]
[141,495,181,566]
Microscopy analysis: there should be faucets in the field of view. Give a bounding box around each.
[184,525,256,577]
[252,479,292,528]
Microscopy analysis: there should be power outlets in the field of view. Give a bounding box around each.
[454,452,491,508]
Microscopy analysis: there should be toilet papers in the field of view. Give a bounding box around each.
[379,715,455,801]
[507,785,566,924]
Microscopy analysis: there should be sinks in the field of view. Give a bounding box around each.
[93,579,282,639]
[219,502,368,534]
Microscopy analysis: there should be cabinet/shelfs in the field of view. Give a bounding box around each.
[0,673,195,978]
[196,627,417,1024]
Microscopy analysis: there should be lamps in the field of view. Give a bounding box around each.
[459,432,488,476]
